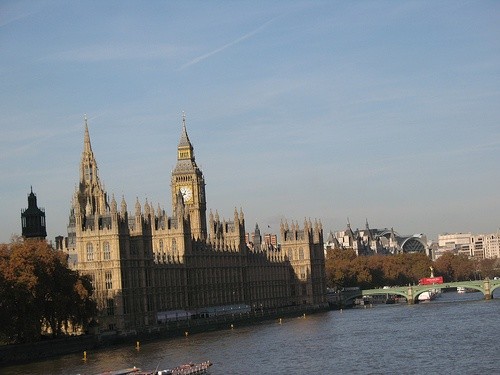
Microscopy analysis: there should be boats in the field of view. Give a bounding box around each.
[97,366,142,375]
[131,360,214,375]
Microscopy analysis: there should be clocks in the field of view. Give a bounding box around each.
[179,186,192,203]
